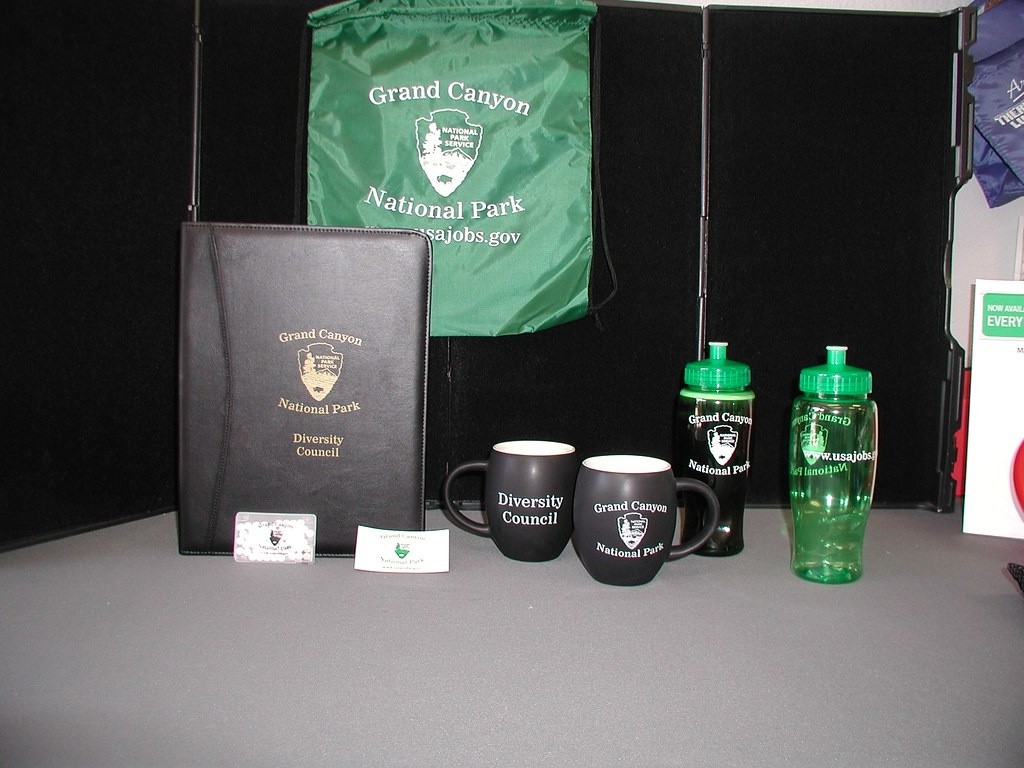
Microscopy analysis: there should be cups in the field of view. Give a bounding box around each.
[442,441,579,563]
[573,455,719,586]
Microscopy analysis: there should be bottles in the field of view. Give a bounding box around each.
[672,343,755,558]
[788,346,879,583]
